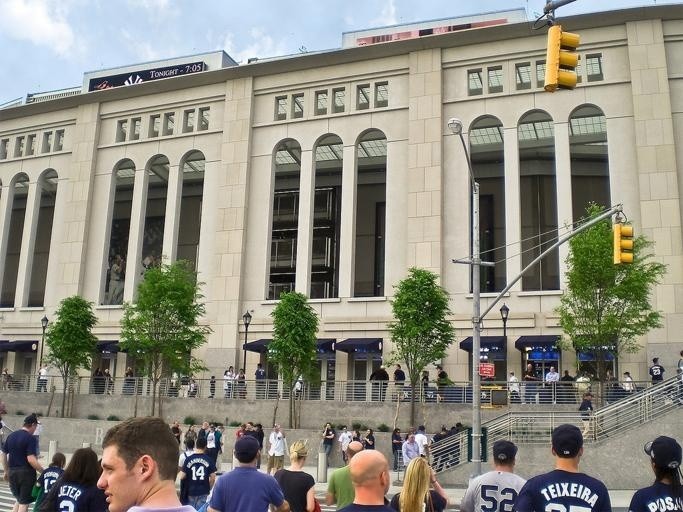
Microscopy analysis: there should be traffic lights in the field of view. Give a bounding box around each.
[491,386,509,407]
[619,225,634,265]
[544,25,580,91]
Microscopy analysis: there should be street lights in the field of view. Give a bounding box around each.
[35,313,49,393]
[445,114,484,480]
[238,307,253,379]
[498,303,510,388]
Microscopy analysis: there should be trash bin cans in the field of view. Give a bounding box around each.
[317,452,328,483]
[48,440,58,465]
[82,442,92,449]
[467,427,488,462]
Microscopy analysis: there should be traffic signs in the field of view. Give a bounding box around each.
[478,363,495,376]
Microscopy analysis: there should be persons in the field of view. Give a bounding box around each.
[107,254,126,305]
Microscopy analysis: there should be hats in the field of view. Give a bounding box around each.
[652,357,659,364]
[493,439,518,462]
[234,435,260,464]
[23,415,40,425]
[585,392,593,396]
[551,423,583,455]
[643,435,682,470]
